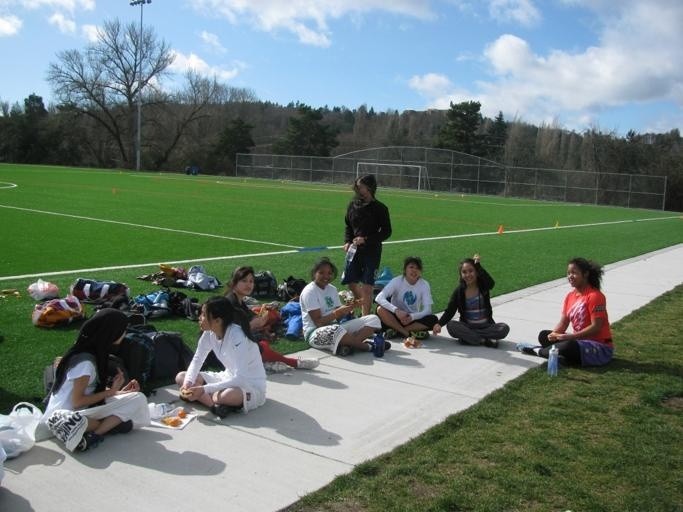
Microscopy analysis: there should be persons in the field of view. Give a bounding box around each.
[300,260,391,357]
[522,257,614,368]
[175,295,267,420]
[375,256,438,340]
[41,308,151,453]
[432,254,510,349]
[223,266,319,374]
[344,174,392,317]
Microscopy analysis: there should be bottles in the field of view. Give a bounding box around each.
[344,242,356,262]
[545,345,559,378]
[154,403,175,417]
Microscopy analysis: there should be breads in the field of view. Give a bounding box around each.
[162,418,178,426]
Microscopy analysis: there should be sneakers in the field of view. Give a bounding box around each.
[294,356,319,370]
[263,359,289,373]
[363,328,396,357]
[408,330,430,339]
[338,345,352,356]
[77,431,102,452]
[110,420,132,435]
[516,342,541,354]
[210,402,228,416]
[485,337,498,348]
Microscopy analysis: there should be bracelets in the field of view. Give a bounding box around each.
[363,236,368,243]
[333,310,338,319]
[106,389,116,398]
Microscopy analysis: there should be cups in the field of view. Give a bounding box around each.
[342,290,354,305]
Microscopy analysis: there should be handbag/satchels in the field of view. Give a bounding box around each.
[121,330,186,391]
[248,270,276,300]
[30,278,201,325]
[276,276,308,301]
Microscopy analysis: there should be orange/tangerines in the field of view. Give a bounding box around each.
[177,410,186,418]
